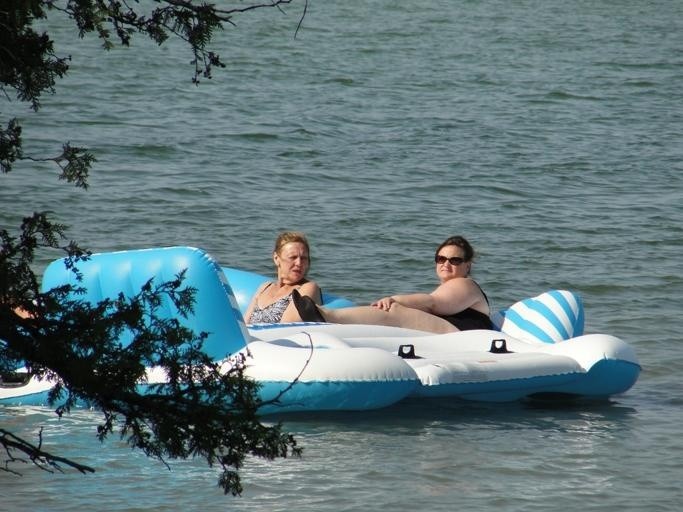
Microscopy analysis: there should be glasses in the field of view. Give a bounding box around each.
[434,256,467,264]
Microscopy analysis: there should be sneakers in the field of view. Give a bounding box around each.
[293,289,327,322]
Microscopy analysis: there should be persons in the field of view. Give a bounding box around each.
[292,236,493,333]
[243,232,322,324]
